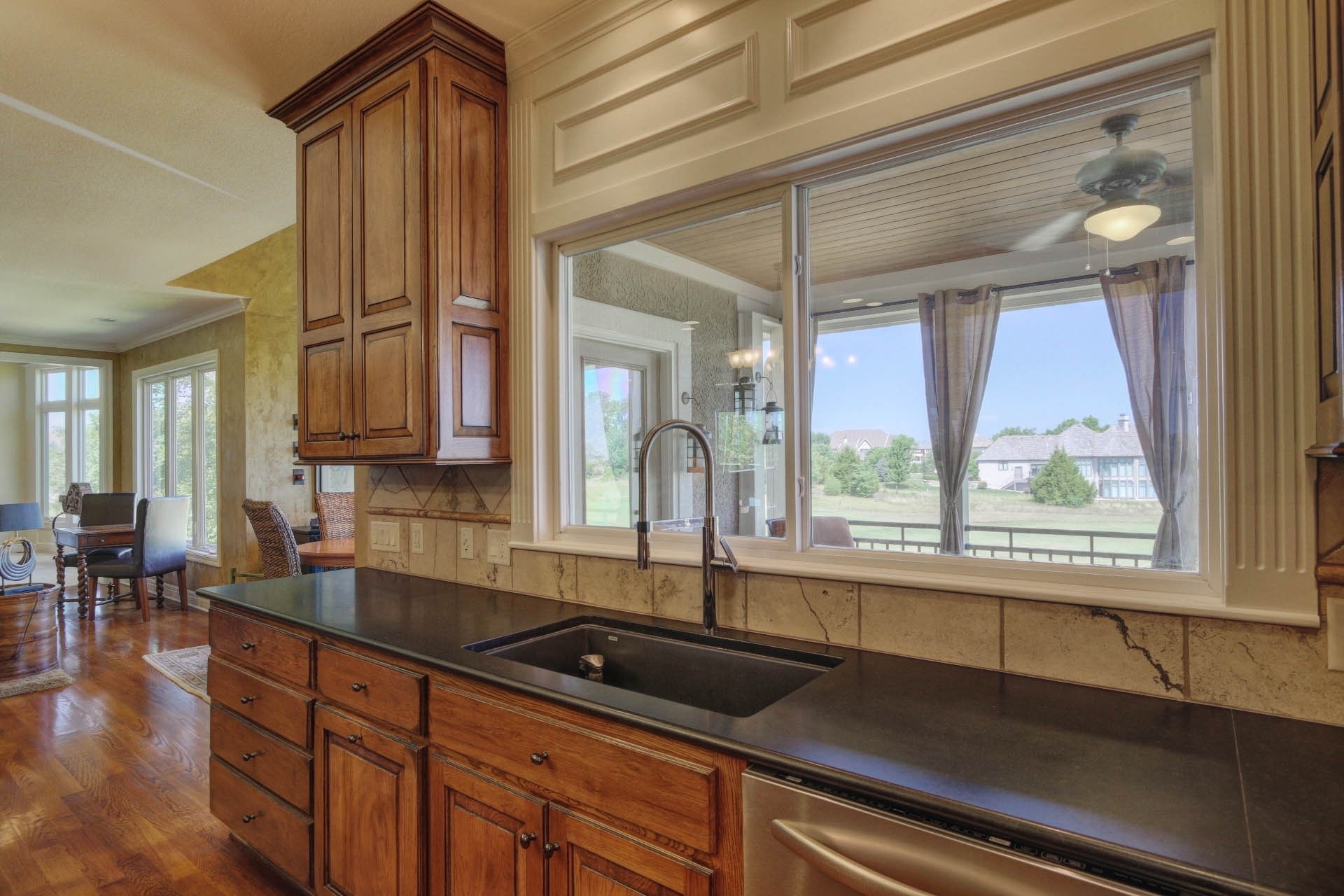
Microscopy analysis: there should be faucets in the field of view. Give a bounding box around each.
[631,416,740,630]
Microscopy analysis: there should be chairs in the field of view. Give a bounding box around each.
[56,492,134,605]
[243,499,302,577]
[771,516,856,550]
[86,496,190,622]
[313,492,358,541]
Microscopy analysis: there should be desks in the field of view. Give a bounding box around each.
[56,523,164,619]
[296,540,356,568]
[289,526,319,543]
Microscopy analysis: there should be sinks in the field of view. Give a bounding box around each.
[456,613,847,721]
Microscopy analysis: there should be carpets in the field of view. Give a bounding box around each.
[141,643,210,710]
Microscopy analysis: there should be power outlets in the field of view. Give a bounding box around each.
[461,528,476,561]
[487,528,511,566]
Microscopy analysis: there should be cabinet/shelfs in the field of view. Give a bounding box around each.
[190,599,744,895]
[264,0,514,469]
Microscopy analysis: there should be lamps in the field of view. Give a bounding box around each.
[1,502,45,544]
[754,371,783,444]
[734,376,755,420]
[681,393,712,473]
[1078,110,1172,244]
[727,347,763,392]
